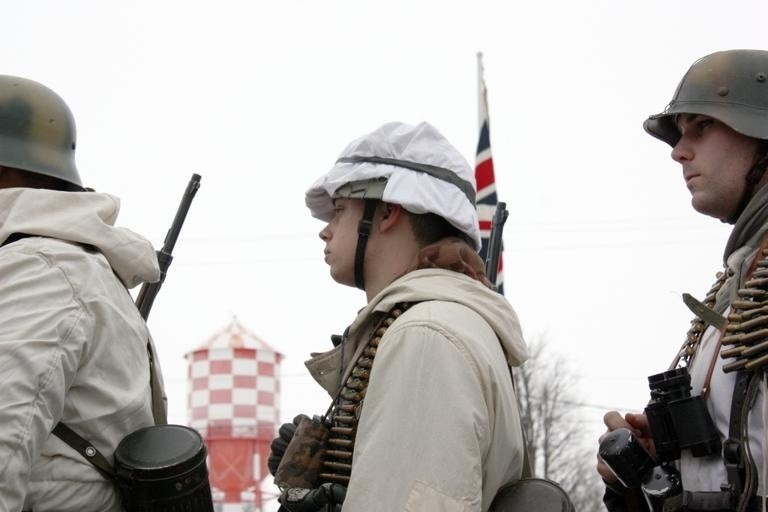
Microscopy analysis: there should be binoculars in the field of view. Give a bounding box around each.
[644,366,721,460]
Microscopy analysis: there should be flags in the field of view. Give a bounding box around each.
[471,87,505,295]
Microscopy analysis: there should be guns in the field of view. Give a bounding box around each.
[135,172,201,320]
[486,203,509,282]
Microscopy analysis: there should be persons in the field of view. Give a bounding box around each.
[595,49,768,512]
[265,120,530,512]
[0,73,166,512]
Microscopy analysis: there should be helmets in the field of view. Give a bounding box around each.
[1,74,89,193]
[643,49,767,148]
[302,120,483,255]
